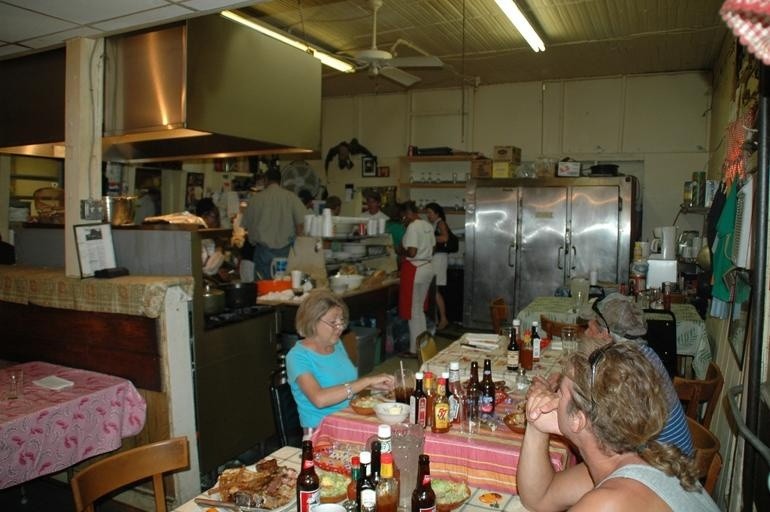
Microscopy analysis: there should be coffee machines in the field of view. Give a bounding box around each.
[648,226,677,261]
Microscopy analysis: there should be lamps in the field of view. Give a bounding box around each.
[220,9,356,74]
[495,0,547,54]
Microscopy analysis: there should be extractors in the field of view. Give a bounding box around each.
[0,15,324,166]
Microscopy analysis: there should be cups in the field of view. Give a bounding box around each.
[270,257,287,279]
[589,271,599,286]
[3,369,26,403]
[561,327,577,358]
[302,208,335,239]
[570,277,590,313]
[678,236,702,258]
[393,369,416,405]
[357,217,386,237]
[290,270,302,289]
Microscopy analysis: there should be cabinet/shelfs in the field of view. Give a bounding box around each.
[9,154,65,208]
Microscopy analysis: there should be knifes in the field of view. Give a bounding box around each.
[460,341,492,351]
[194,498,273,511]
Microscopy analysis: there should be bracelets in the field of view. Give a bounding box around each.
[344,384,353,401]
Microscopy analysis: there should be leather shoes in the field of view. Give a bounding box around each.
[438,320,448,329]
[397,352,418,359]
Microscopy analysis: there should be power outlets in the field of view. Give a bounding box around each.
[51,182,59,188]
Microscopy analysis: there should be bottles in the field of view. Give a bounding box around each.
[619,274,673,312]
[684,180,699,207]
[408,358,497,433]
[507,319,543,371]
[295,438,321,512]
[418,170,471,212]
[633,242,642,262]
[514,366,530,399]
[345,422,435,512]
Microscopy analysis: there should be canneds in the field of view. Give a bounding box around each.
[359,223,365,236]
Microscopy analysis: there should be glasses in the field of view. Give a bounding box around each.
[320,319,347,329]
[592,295,610,334]
[589,342,616,394]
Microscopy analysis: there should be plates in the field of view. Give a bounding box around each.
[323,243,367,262]
[370,387,396,402]
[210,466,297,511]
[309,443,364,512]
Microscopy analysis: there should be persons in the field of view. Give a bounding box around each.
[238,168,308,278]
[197,197,221,228]
[299,189,448,358]
[516,341,722,512]
[525,292,694,458]
[284,288,396,438]
[133,187,161,224]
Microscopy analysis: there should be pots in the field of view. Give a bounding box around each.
[102,195,137,226]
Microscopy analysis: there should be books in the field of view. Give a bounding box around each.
[32,375,74,390]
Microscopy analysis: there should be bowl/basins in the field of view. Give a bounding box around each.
[199,287,226,317]
[328,282,349,294]
[218,282,258,309]
[590,164,620,177]
[494,389,509,407]
[332,217,355,238]
[509,333,552,350]
[432,478,472,511]
[33,186,66,217]
[371,403,411,425]
[349,395,385,416]
[326,273,365,290]
[504,412,527,436]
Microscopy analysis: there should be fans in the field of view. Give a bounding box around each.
[323,2,444,85]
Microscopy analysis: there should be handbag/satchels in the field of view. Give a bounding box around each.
[433,231,459,252]
